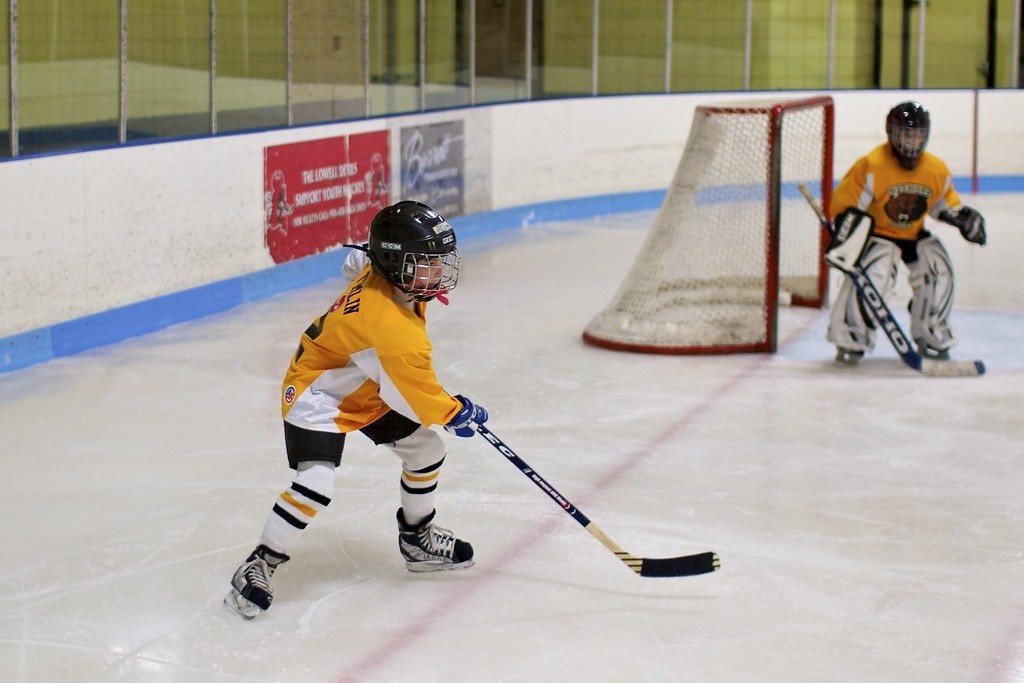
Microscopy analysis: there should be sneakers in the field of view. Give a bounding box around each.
[836,346,865,366]
[223,544,291,621]
[914,336,951,360]
[395,507,474,572]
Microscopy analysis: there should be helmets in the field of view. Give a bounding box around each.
[886,102,929,169]
[369,200,460,302]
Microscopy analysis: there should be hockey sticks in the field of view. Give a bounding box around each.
[796,181,990,379]
[476,422,725,581]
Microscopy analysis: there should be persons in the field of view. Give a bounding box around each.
[824,101,987,357]
[230,200,489,611]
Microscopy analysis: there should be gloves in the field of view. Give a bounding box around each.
[954,207,986,246]
[444,394,488,439]
